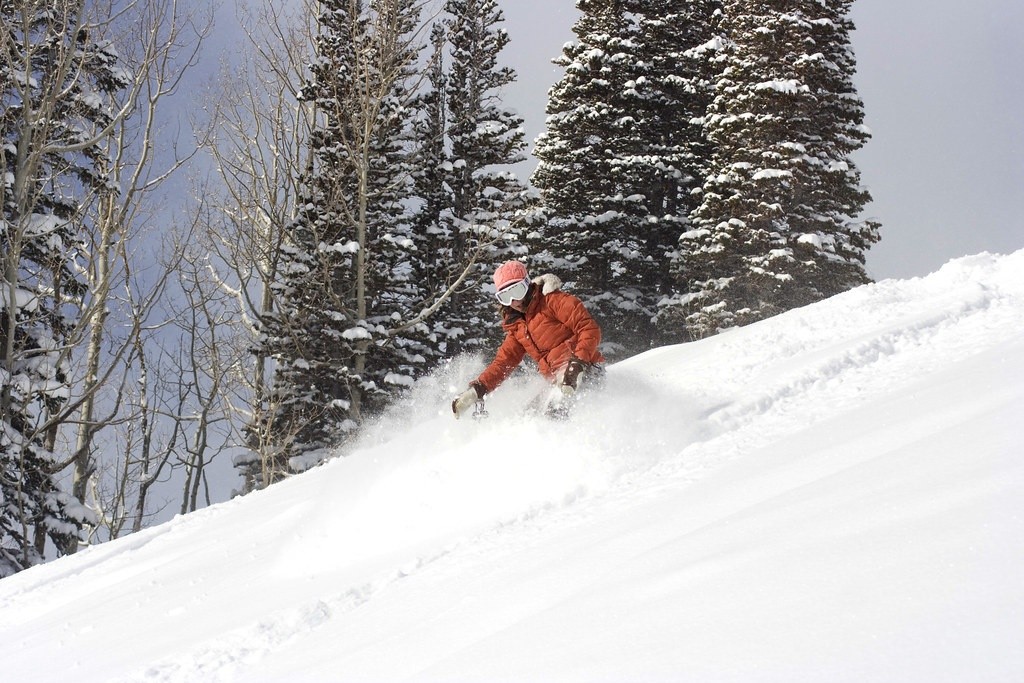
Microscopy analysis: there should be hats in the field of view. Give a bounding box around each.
[494,260,527,291]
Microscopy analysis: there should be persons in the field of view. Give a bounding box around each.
[452,260,604,425]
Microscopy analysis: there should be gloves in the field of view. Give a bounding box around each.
[561,356,589,399]
[452,378,487,420]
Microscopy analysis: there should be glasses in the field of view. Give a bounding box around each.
[495,274,530,306]
